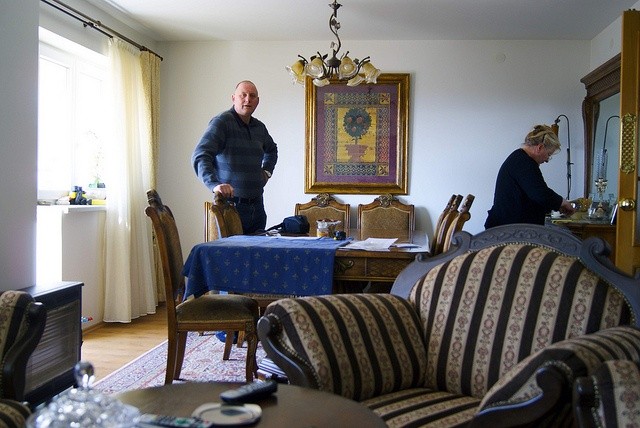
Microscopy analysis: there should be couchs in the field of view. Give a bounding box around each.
[0,290,47,428]
[572,358,640,428]
[254,226,637,428]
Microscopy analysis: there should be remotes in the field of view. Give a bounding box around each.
[218,378,274,402]
[133,410,210,428]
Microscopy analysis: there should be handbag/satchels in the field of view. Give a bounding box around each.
[282,215,309,234]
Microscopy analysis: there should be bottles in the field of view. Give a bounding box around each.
[79,187,83,199]
[70,186,79,205]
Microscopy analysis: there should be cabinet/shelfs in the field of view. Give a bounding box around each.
[36,205,106,337]
[1,281,84,413]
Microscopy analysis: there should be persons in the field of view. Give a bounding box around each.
[195,81,278,342]
[484,124,575,229]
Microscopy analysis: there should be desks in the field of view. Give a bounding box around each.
[180,226,429,297]
[544,210,614,275]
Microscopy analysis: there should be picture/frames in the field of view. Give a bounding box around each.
[305,72,410,195]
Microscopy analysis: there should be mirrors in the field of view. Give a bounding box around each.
[580,50,620,226]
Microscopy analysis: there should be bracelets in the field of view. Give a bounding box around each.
[265,170,272,178]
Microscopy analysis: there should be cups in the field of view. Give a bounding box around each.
[317,228,328,238]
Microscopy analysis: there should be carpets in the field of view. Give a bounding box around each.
[88,327,290,402]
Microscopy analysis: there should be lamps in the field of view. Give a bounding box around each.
[283,0,381,87]
[591,115,619,220]
[550,115,574,201]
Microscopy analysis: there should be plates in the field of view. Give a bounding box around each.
[193,400,264,427]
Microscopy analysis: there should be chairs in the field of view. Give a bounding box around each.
[357,193,414,243]
[439,194,474,253]
[433,196,463,255]
[204,200,297,359]
[295,193,350,239]
[430,195,456,252]
[145,189,260,385]
[215,191,243,236]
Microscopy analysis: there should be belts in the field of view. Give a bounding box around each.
[233,197,262,203]
